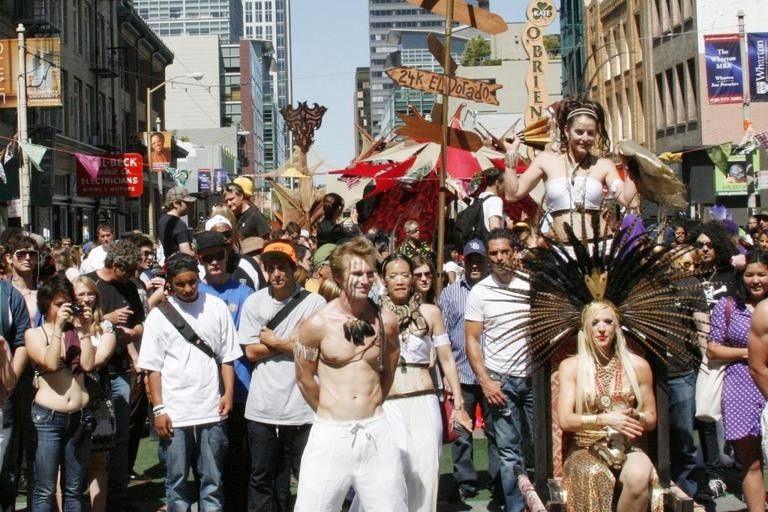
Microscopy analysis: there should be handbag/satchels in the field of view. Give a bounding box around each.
[695,352,726,423]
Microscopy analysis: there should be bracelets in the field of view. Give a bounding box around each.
[153,404,164,411]
[52,332,61,339]
[639,412,646,424]
[582,414,601,430]
[154,408,166,417]
[79,333,90,339]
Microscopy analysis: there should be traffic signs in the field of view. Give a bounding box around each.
[384,67,502,105]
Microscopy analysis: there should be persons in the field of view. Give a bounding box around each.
[144,132,170,170]
[0,183,768,512]
[502,100,638,263]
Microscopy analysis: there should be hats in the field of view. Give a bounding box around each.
[164,187,198,204]
[262,243,296,268]
[196,215,234,252]
[461,238,485,257]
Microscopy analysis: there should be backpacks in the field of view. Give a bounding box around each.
[455,194,498,254]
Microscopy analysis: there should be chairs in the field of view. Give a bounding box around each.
[514,256,696,512]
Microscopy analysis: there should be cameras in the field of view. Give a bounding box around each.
[67,304,84,317]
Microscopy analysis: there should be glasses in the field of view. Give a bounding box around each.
[143,250,154,257]
[15,252,37,261]
[694,241,714,249]
[202,251,224,263]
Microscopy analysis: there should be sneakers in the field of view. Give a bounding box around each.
[694,479,726,501]
[445,487,474,503]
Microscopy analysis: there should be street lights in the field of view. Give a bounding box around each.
[146,72,205,237]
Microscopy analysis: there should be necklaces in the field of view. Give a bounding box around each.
[593,356,618,407]
[333,301,375,347]
